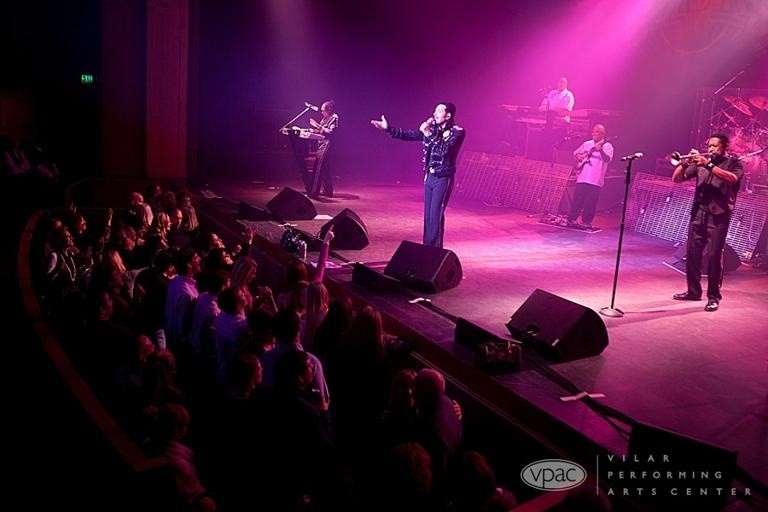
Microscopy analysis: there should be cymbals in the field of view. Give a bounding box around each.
[747,96,768,113]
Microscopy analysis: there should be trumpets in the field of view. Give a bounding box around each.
[669,152,713,166]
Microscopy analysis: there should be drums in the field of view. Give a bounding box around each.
[757,127,768,148]
[722,95,752,123]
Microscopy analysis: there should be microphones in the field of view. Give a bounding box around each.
[302,101,319,112]
[425,117,435,125]
[619,152,643,162]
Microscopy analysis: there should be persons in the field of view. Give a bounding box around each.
[672,133,744,311]
[0,78,615,511]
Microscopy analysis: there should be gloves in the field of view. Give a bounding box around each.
[324,225,335,245]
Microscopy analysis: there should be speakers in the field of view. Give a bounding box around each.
[504,288,608,362]
[673,238,741,275]
[266,185,316,220]
[384,240,462,293]
[319,208,368,251]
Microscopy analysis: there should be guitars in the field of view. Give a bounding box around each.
[575,136,618,170]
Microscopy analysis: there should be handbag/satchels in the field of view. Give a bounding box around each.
[477,342,522,372]
[280,231,314,253]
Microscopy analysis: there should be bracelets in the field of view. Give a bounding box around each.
[704,161,714,171]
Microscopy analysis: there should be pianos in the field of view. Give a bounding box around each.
[282,126,325,140]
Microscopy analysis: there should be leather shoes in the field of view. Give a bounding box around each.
[705,299,719,311]
[673,291,699,300]
[320,191,333,197]
